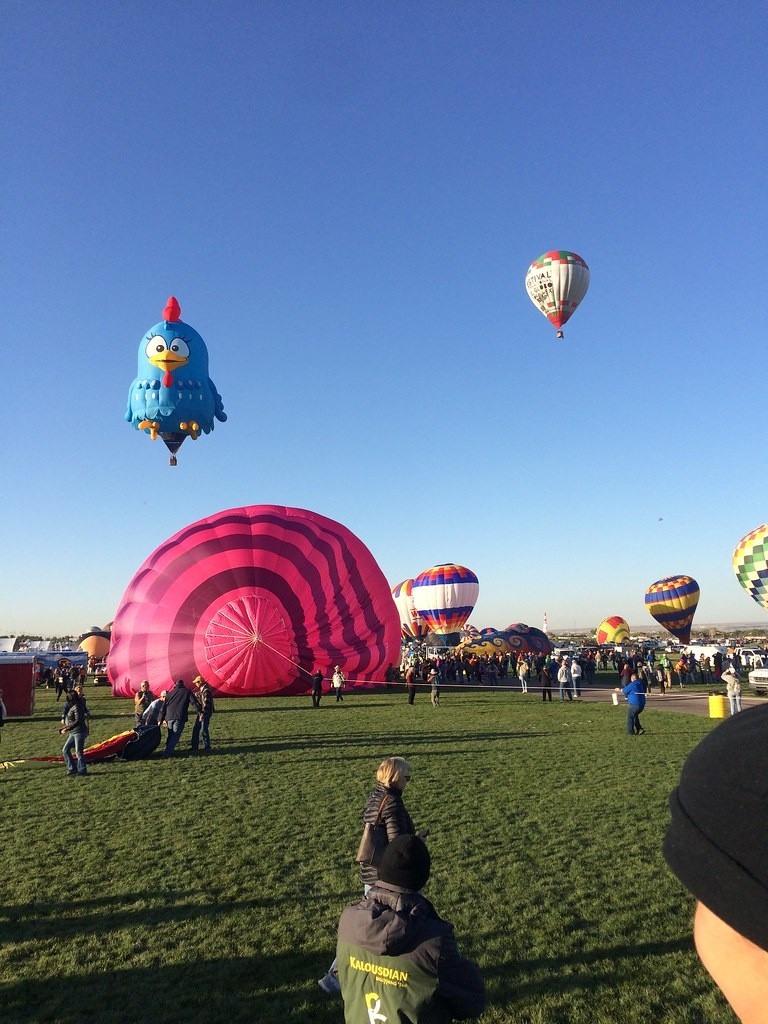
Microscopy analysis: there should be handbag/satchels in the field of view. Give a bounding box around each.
[355,793,391,869]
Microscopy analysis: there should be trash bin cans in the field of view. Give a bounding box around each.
[708,690,727,719]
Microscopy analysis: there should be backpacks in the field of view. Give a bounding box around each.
[434,675,440,685]
[732,679,742,694]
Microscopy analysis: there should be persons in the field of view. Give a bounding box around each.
[327,834,486,1024]
[427,669,442,707]
[39,655,111,702]
[405,667,418,706]
[189,676,216,751]
[330,665,346,702]
[134,680,160,727]
[158,679,203,760]
[57,686,92,735]
[385,629,768,702]
[59,691,91,778]
[310,669,323,708]
[615,674,648,735]
[317,755,430,997]
[720,666,743,716]
[663,704,768,1024]
[141,690,170,728]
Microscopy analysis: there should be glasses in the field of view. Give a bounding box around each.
[404,776,410,782]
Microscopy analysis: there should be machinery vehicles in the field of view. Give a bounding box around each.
[93,662,111,686]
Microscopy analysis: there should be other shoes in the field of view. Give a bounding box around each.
[635,728,644,735]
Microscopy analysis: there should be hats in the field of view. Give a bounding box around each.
[379,833,431,893]
[334,665,340,669]
[430,668,437,674]
[192,676,205,683]
[161,690,169,697]
[729,668,735,673]
[141,680,149,686]
[662,701,768,954]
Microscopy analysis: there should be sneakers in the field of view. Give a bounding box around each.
[318,974,341,996]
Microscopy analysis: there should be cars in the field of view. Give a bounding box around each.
[552,642,659,664]
[665,646,680,653]
[733,648,768,666]
[748,654,768,697]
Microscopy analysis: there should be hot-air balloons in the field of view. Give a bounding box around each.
[389,561,480,648]
[123,294,227,468]
[594,616,633,647]
[644,574,700,645]
[524,249,591,340]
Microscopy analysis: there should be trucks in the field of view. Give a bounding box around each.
[420,646,450,662]
